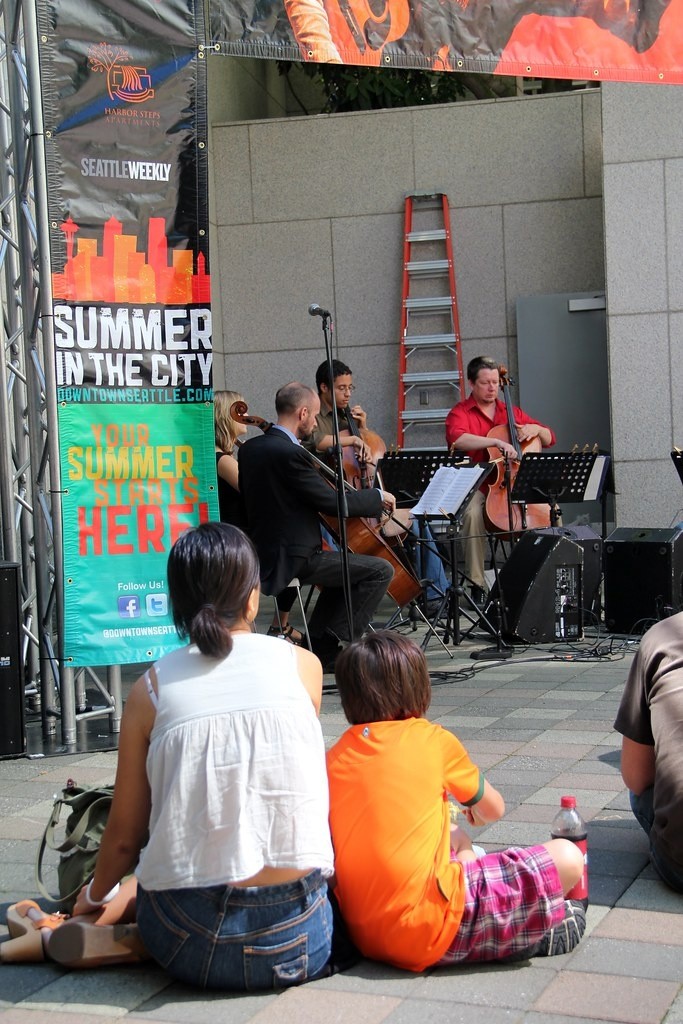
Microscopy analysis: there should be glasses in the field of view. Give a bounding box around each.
[335,386,356,393]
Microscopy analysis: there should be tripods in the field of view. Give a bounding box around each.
[373,450,478,630]
[413,461,508,657]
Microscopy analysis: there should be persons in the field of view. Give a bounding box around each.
[324,626,590,972]
[236,382,397,676]
[211,389,307,646]
[445,357,558,605]
[0,522,336,990]
[306,355,457,625]
[608,605,683,893]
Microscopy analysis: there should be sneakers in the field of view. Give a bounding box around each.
[469,585,486,611]
[509,896,586,961]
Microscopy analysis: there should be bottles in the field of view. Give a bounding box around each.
[550,796,589,915]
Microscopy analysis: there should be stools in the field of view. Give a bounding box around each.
[250,577,314,650]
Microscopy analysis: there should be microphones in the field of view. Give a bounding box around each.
[308,303,330,317]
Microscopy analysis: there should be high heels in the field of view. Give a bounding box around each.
[0,899,70,963]
[52,922,154,968]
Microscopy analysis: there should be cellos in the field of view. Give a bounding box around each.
[229,398,423,609]
[483,363,563,543]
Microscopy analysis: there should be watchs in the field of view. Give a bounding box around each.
[87,876,123,907]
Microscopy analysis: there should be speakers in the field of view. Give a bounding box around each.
[0,560,26,761]
[531,524,603,623]
[605,527,682,634]
[480,531,584,646]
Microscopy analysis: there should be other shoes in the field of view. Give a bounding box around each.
[420,599,464,622]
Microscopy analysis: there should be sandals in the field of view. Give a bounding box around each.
[267,626,300,646]
[287,623,305,643]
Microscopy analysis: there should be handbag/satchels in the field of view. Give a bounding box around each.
[35,785,148,918]
